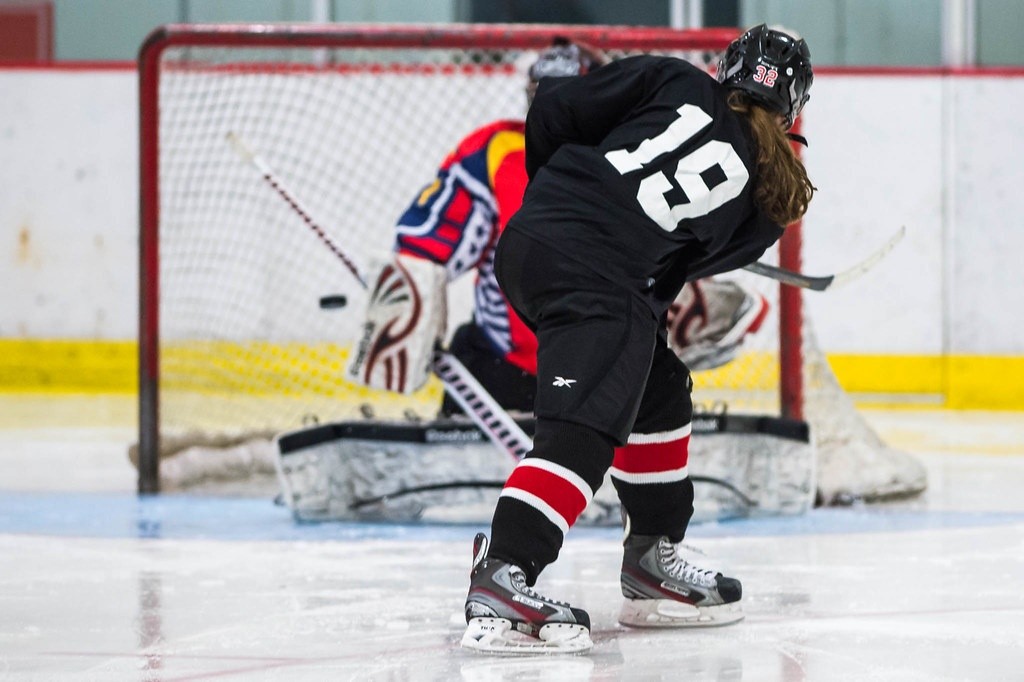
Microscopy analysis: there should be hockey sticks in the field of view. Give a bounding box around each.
[224,127,611,530]
[739,223,911,293]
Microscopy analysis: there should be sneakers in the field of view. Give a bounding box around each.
[616,503,743,626]
[459,534,595,653]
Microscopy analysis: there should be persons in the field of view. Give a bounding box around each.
[274,39,769,520]
[458,23,814,660]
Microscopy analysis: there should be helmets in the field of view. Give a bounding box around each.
[717,23,813,145]
[526,42,608,111]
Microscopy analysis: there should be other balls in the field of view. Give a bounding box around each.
[318,294,346,310]
